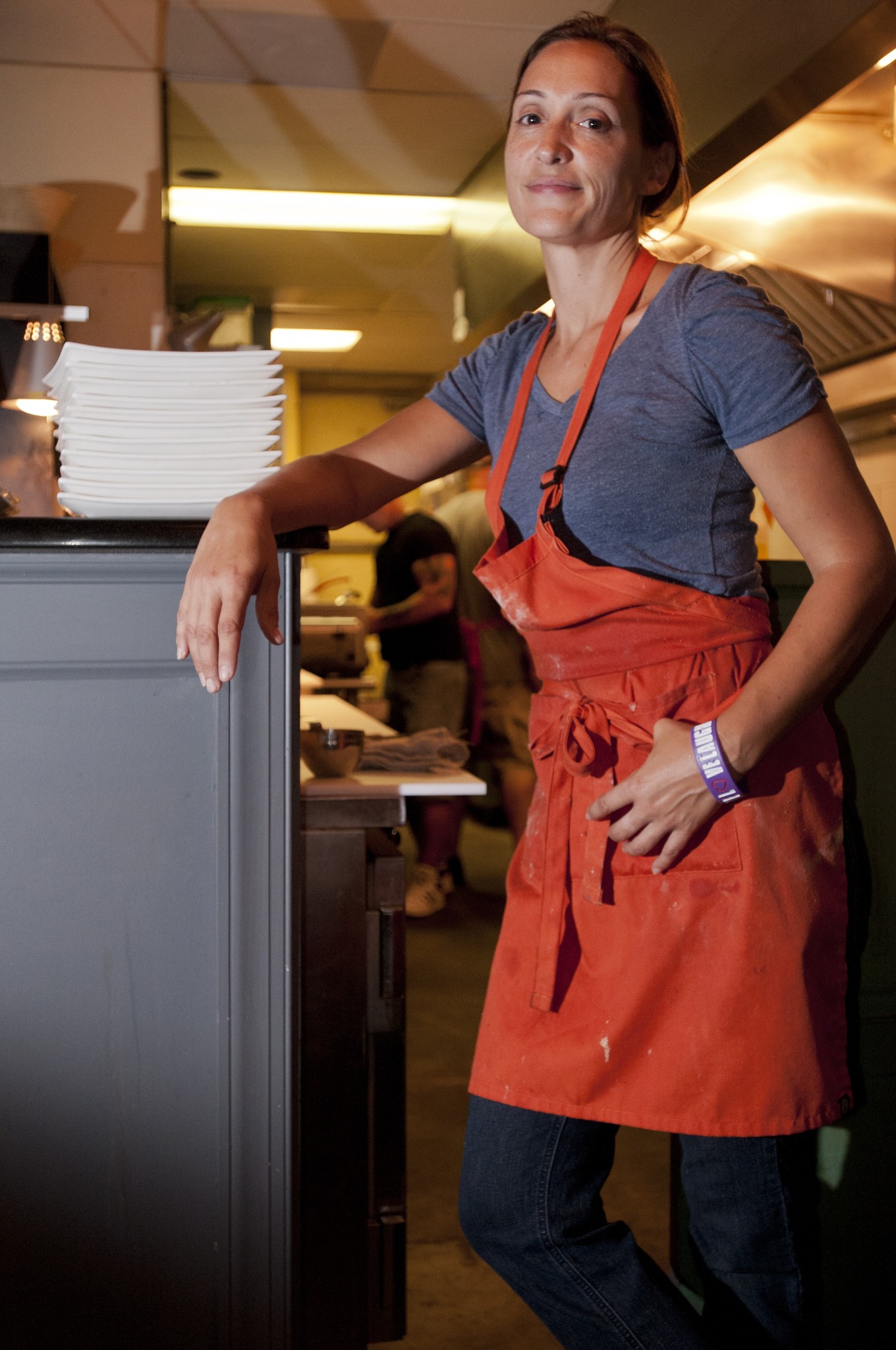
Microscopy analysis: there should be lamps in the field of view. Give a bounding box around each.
[0,316,74,420]
[263,300,364,362]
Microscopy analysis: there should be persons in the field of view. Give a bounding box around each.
[345,494,472,919]
[178,8,896,1350]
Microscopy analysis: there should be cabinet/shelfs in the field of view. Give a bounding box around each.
[2,512,335,1350]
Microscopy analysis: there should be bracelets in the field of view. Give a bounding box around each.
[690,715,747,806]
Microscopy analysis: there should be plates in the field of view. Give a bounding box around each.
[41,342,287,519]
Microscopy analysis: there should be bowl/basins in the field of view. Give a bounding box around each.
[299,721,365,778]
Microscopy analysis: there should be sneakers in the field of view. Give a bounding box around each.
[403,862,467,919]
